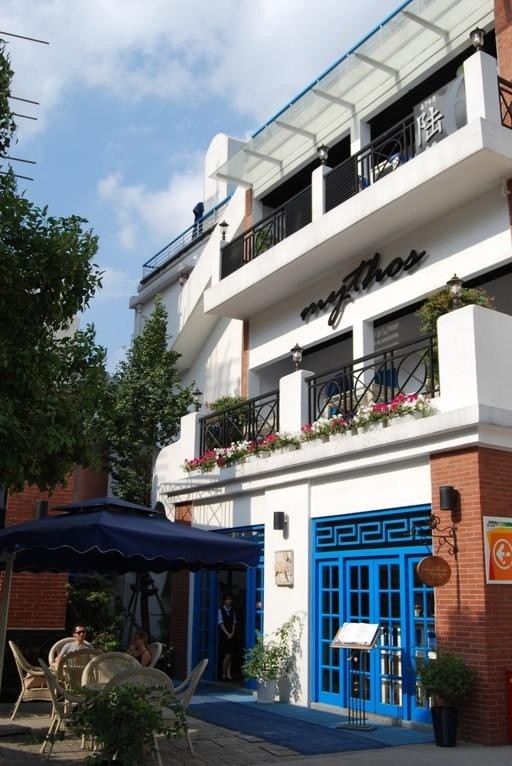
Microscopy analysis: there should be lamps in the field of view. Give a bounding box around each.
[447,274,462,308]
[219,219,229,239]
[317,143,330,163]
[439,485,458,511]
[469,26,486,50]
[290,343,304,371]
[273,512,287,530]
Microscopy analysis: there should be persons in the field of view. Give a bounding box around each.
[125,629,152,667]
[217,592,238,680]
[55,623,95,667]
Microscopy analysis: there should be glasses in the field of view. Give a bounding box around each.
[75,630,87,635]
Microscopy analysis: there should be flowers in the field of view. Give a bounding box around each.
[298,390,430,441]
[182,430,301,473]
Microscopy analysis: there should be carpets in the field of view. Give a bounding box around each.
[166,678,437,755]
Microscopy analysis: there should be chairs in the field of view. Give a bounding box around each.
[7,638,209,766]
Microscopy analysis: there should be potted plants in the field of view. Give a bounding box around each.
[418,652,478,748]
[242,610,300,705]
